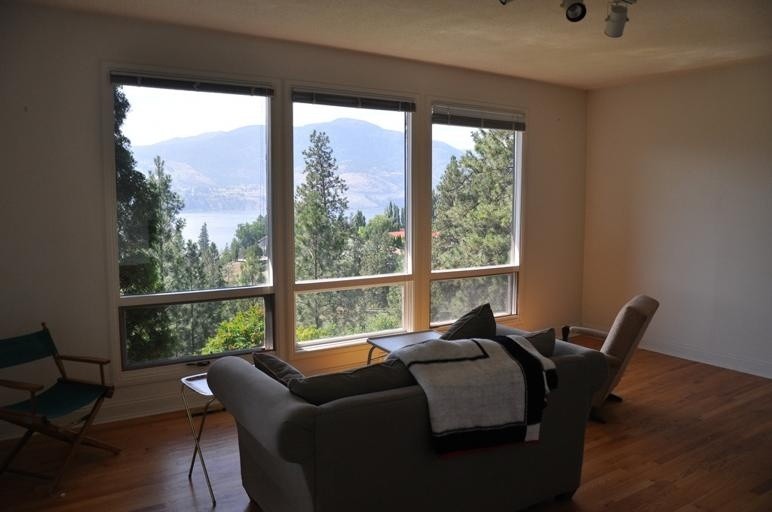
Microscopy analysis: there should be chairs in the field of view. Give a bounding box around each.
[560,293,660,424]
[0,321,123,499]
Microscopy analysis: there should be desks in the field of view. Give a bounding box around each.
[178,372,218,504]
[366,327,443,367]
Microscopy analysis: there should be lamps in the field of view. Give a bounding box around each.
[603,0,636,38]
[560,0,586,23]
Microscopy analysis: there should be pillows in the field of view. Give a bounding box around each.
[250,350,411,407]
[439,302,555,358]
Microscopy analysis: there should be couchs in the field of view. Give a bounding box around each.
[205,320,625,512]
